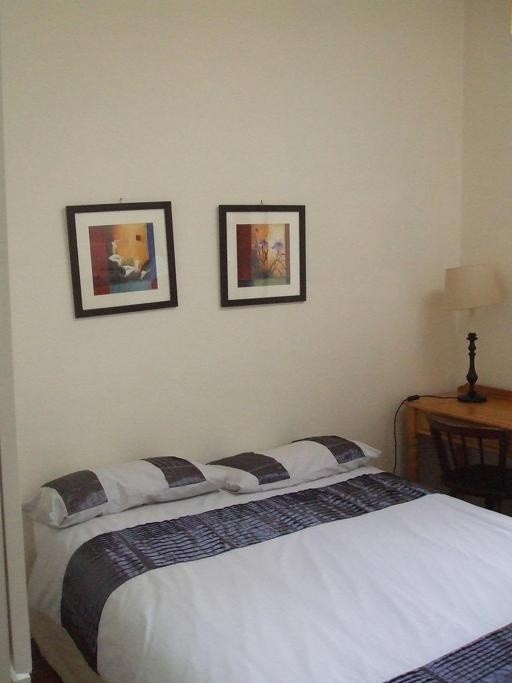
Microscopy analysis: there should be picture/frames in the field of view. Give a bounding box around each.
[65,199,177,320]
[218,202,307,307]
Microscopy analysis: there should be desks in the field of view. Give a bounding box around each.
[398,383,512,495]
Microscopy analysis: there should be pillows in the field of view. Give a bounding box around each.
[23,452,230,530]
[208,433,381,495]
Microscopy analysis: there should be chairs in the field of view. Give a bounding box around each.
[423,413,512,517]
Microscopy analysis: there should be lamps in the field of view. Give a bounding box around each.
[441,259,502,405]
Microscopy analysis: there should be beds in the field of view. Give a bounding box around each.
[29,435,512,682]
